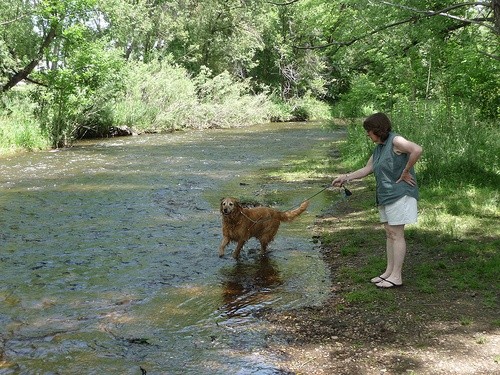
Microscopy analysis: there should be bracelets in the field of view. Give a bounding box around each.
[404,168,409,173]
[345,174,349,182]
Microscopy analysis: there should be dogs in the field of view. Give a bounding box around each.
[219,196,310,258]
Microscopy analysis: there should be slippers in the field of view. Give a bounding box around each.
[370,276,404,288]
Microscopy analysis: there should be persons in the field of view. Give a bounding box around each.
[330,112,423,288]
